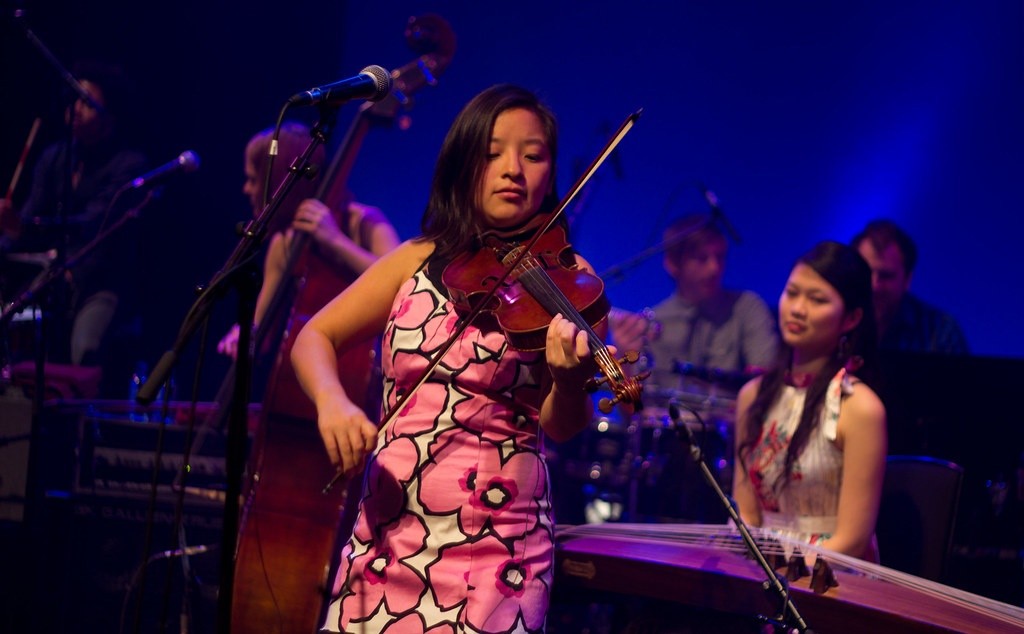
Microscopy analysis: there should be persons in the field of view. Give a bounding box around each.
[288,84,617,634]
[4,60,143,363]
[214,124,404,634]
[854,225,914,344]
[622,241,891,633]
[607,212,779,461]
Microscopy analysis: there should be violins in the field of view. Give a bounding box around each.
[440,210,646,418]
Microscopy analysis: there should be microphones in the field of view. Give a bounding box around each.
[669,401,699,461]
[130,151,200,190]
[289,65,393,111]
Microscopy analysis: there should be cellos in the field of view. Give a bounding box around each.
[233,15,456,634]
